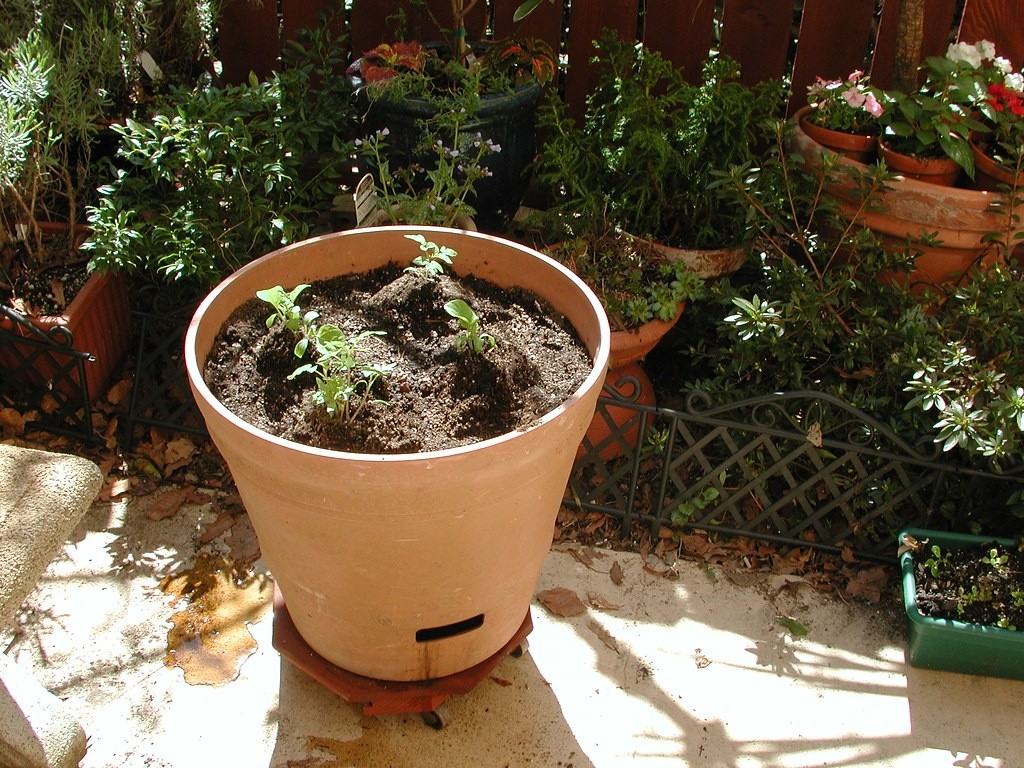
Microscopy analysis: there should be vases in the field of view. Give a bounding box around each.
[355,201,478,232]
[941,110,981,137]
[803,115,877,163]
[784,105,1024,319]
[967,130,1024,192]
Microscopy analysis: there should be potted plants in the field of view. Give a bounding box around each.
[517,27,793,289]
[876,56,997,187]
[898,528,1024,681]
[346,0,557,234]
[0,0,133,405]
[183,224,613,718]
[61,0,264,199]
[537,221,706,371]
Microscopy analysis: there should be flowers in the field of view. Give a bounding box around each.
[920,39,1024,94]
[986,84,1024,167]
[350,61,502,229]
[806,68,907,133]
